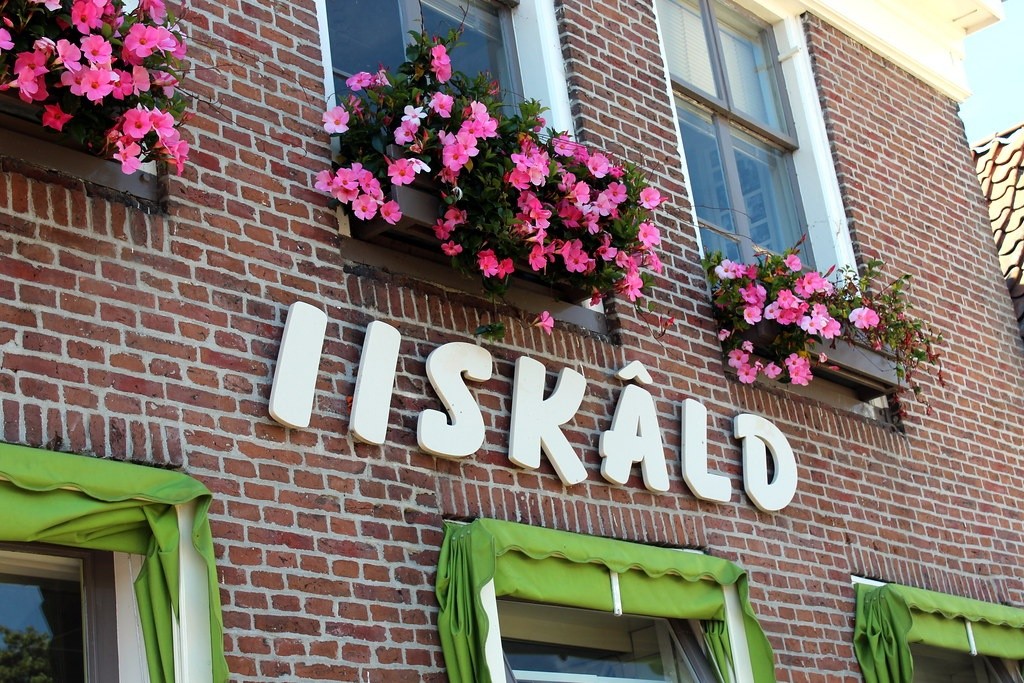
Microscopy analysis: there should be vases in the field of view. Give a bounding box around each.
[739,307,912,398]
[352,143,594,304]
[0,79,88,150]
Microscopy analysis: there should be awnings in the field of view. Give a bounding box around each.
[854,583,1024,683]
[435,518,775,683]
[1,440,230,683]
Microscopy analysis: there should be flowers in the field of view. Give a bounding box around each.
[0,0,195,180]
[313,0,679,340]
[700,231,948,417]
